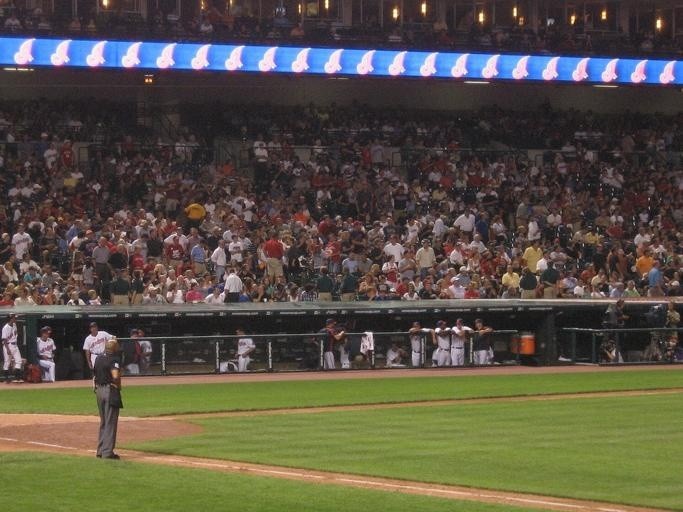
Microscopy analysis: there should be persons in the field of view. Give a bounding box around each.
[91,340,123,458]
[1,0,683,380]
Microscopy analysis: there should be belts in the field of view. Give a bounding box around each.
[91,353,100,355]
[4,342,16,345]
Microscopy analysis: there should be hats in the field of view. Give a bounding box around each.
[451,277,460,284]
[7,313,17,320]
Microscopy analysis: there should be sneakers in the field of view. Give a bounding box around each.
[10,379,25,383]
[95,452,119,460]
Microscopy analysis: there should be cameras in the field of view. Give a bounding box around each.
[600,344,613,353]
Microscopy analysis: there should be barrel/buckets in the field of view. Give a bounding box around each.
[519,331,536,356]
[511,333,519,354]
